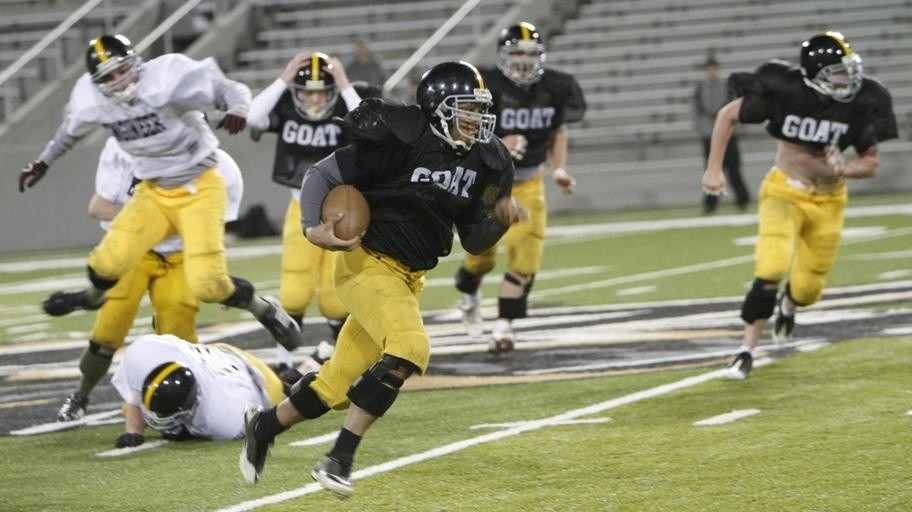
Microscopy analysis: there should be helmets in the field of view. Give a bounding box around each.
[87,34,134,79]
[293,52,335,92]
[416,61,487,129]
[141,362,194,417]
[498,22,541,53]
[800,31,852,79]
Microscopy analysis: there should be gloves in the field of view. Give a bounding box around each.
[19,160,48,192]
[217,112,245,134]
[117,433,143,448]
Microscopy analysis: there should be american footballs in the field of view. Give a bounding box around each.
[321,186,370,241]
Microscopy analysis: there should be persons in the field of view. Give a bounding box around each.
[457,20,588,362]
[56,131,245,423]
[114,335,355,451]
[700,30,901,383]
[19,35,302,428]
[691,56,750,215]
[345,37,385,99]
[238,61,529,497]
[250,50,382,363]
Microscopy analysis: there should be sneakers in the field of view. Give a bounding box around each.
[44,290,105,315]
[240,405,266,484]
[463,294,514,354]
[726,352,751,379]
[311,456,352,500]
[57,394,88,422]
[258,295,341,370]
[772,294,794,342]
